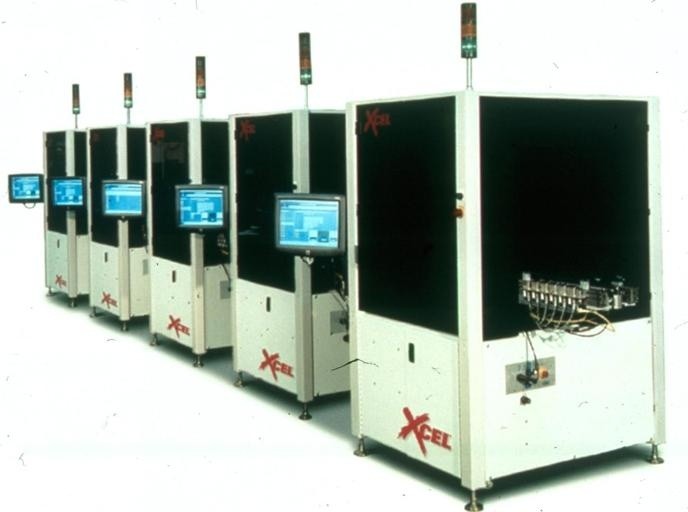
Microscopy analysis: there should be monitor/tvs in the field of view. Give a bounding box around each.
[10,174,43,202]
[101,180,146,219]
[175,184,229,231]
[274,193,346,254]
[50,176,85,209]
